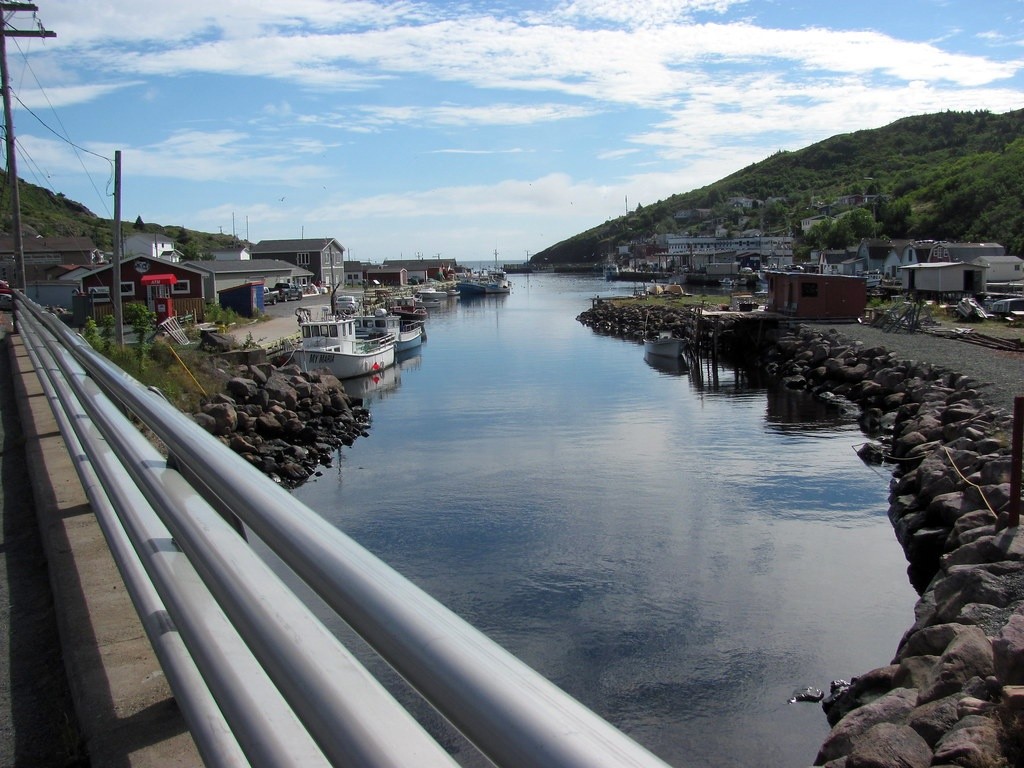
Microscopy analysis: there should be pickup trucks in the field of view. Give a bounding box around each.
[264,286,280,305]
[268,282,303,302]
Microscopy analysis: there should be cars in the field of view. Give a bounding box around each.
[335,296,359,315]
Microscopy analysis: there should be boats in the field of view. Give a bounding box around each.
[340,345,422,408]
[643,325,687,358]
[293,306,397,379]
[353,266,513,352]
[605,263,621,281]
